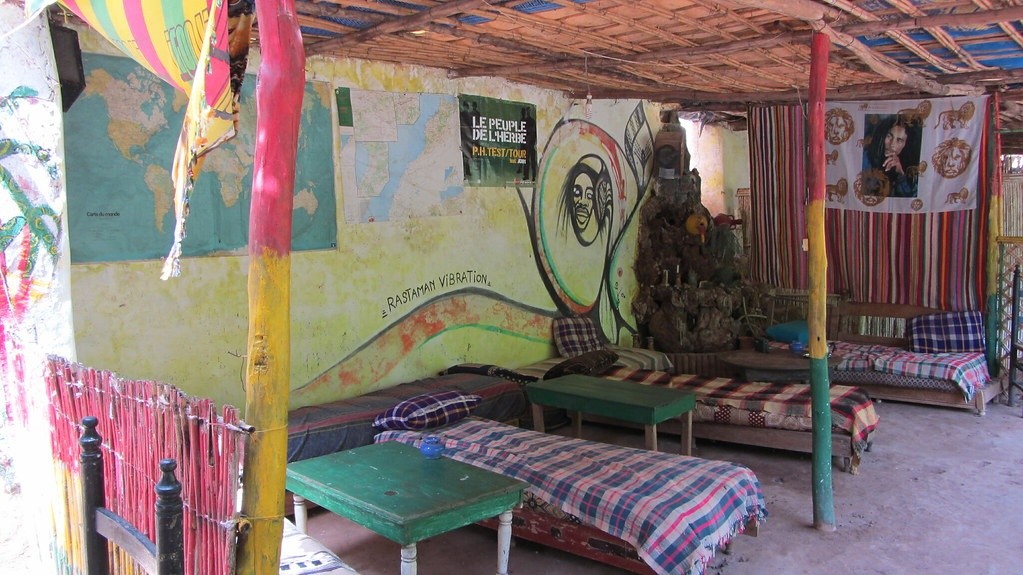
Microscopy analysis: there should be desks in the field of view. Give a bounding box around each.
[525,374,698,457]
[285,440,532,575]
[716,349,844,384]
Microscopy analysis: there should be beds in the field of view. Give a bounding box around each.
[235,463,362,575]
[567,365,880,475]
[766,339,1009,417]
[372,415,768,575]
[241,371,525,516]
[437,343,675,431]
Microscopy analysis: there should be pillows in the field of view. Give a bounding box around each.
[543,349,620,381]
[373,390,484,431]
[552,316,606,359]
[906,309,987,353]
[766,319,809,345]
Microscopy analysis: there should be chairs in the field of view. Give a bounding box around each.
[1007,265,1023,418]
[79,416,184,575]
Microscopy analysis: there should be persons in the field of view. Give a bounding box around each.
[863,117,917,198]
